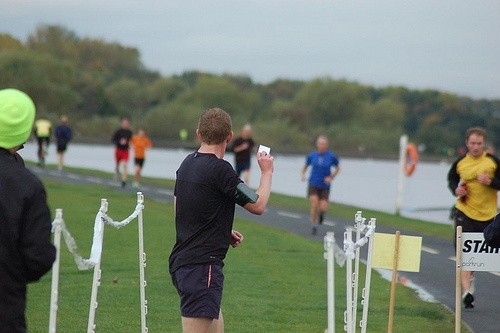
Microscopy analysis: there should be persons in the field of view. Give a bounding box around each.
[168,107,276,333]
[34,114,51,168]
[0,88,57,333]
[355,139,497,158]
[484,213,500,250]
[231,124,259,186]
[300,135,342,236]
[54,115,72,172]
[447,126,500,309]
[111,119,133,188]
[127,126,153,189]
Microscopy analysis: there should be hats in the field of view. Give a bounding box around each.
[0,88,36,150]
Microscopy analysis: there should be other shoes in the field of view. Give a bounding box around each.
[463,291,474,309]
